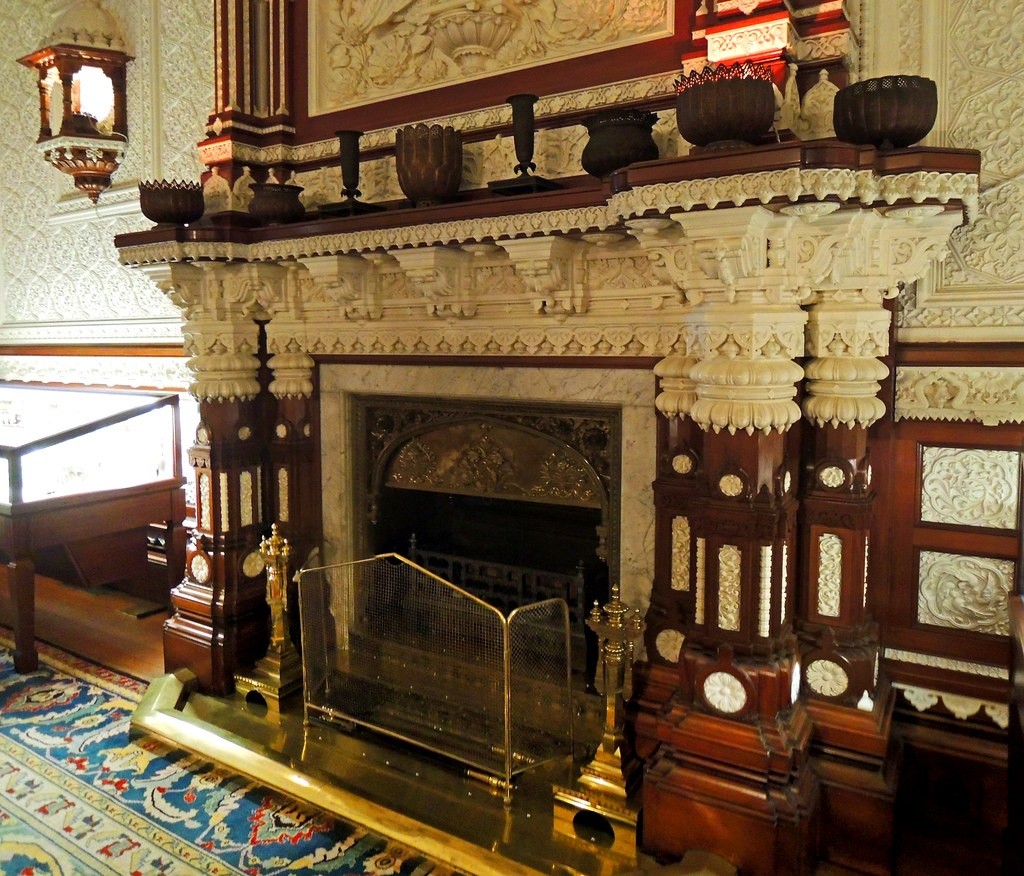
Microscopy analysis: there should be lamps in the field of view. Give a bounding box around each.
[15,2,137,205]
